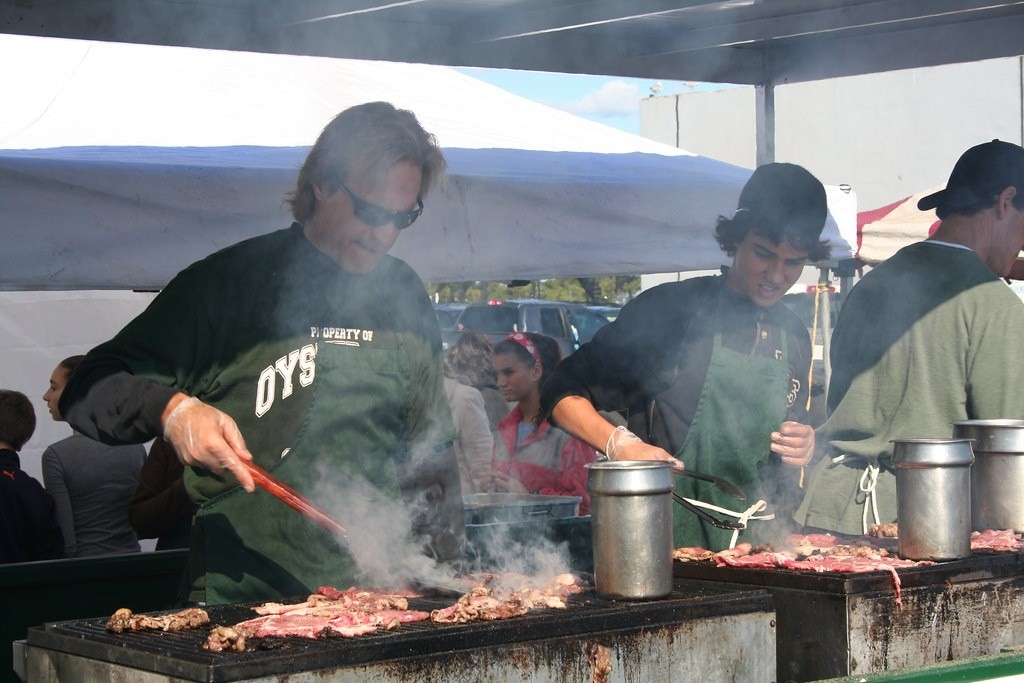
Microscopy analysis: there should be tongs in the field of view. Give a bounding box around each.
[672,469,747,529]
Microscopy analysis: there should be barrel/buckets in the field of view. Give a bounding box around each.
[888,438,977,561]
[950,419,1024,535]
[583,460,676,601]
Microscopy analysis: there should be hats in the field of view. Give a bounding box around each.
[918,138,1024,215]
[740,163,827,237]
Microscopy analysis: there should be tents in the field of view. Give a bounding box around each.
[0,32,853,388]
[840,183,1024,305]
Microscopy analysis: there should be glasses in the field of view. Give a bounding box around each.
[334,176,425,229]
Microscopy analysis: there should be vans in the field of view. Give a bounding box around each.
[435,299,622,361]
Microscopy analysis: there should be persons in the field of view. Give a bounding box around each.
[792,137,1024,535]
[445,332,596,515]
[58,101,464,600]
[540,163,828,550]
[0,390,57,564]
[128,436,195,551]
[41,356,146,558]
